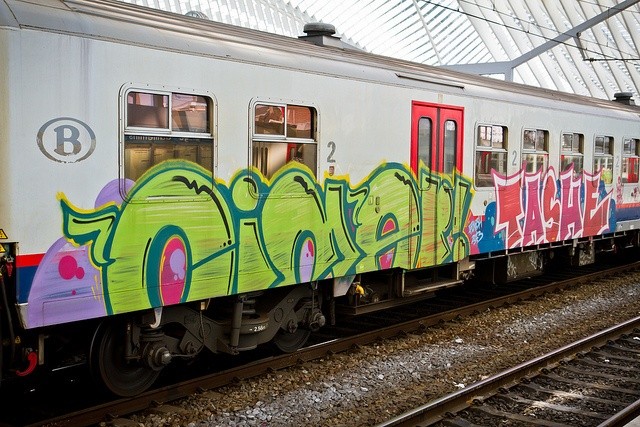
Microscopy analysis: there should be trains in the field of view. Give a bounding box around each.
[0,0,640,398]
